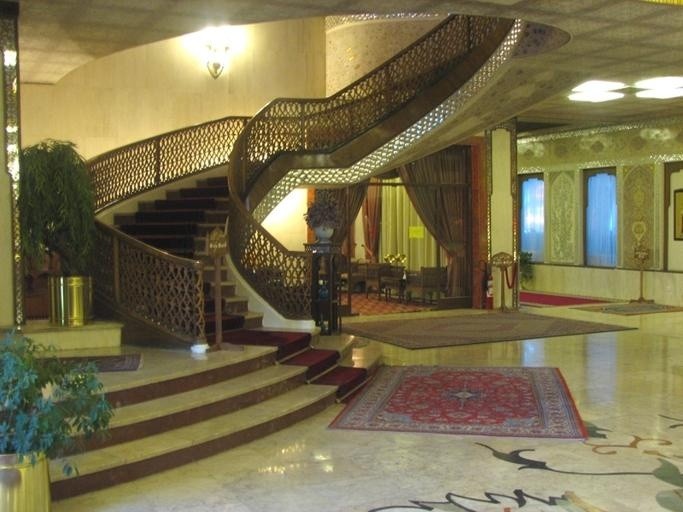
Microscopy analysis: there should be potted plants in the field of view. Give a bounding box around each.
[0,328,108,512]
[304,194,347,243]
[19,141,101,327]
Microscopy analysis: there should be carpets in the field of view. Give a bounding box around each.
[336,309,642,350]
[568,303,683,315]
[325,362,586,438]
[489,290,611,310]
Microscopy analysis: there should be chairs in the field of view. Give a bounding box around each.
[347,255,448,309]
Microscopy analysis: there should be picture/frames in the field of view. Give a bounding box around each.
[673,189,683,241]
